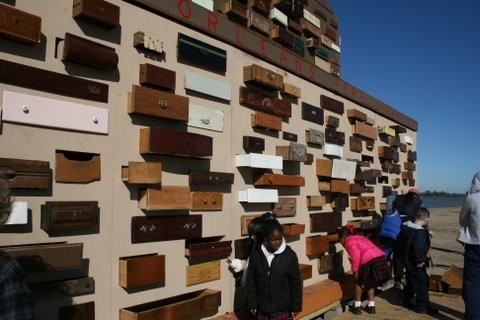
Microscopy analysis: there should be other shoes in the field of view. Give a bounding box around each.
[396,282,404,290]
[417,305,440,314]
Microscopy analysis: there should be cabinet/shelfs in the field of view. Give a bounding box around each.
[0,1,419,320]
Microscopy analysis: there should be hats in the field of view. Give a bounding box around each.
[409,188,420,196]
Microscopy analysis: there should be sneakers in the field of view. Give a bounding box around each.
[364,304,375,314]
[352,303,361,314]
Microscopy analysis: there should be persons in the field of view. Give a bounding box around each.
[456,170,480,320]
[400,206,439,314]
[379,188,434,289]
[338,225,387,315]
[227,212,303,320]
[0,173,35,320]
